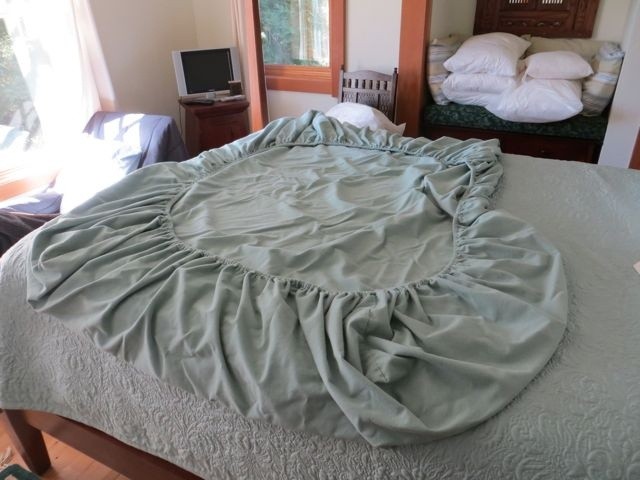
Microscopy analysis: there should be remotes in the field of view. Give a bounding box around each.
[184,100,214,105]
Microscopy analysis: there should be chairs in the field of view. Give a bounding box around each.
[0,113,186,231]
[338,64,398,123]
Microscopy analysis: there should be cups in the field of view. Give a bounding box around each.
[228,81,242,96]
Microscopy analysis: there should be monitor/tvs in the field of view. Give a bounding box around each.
[171,47,235,102]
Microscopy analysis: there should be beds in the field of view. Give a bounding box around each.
[0,108,640,479]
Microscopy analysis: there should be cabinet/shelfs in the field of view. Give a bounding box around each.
[179,99,250,156]
[420,122,601,164]
[473,0,600,38]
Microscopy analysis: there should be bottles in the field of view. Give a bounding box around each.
[207,89,216,99]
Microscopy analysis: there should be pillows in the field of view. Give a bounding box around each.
[443,60,522,92]
[484,79,583,124]
[526,36,598,58]
[443,32,531,76]
[523,51,594,79]
[427,33,462,105]
[326,102,406,135]
[440,85,499,106]
[580,42,624,116]
